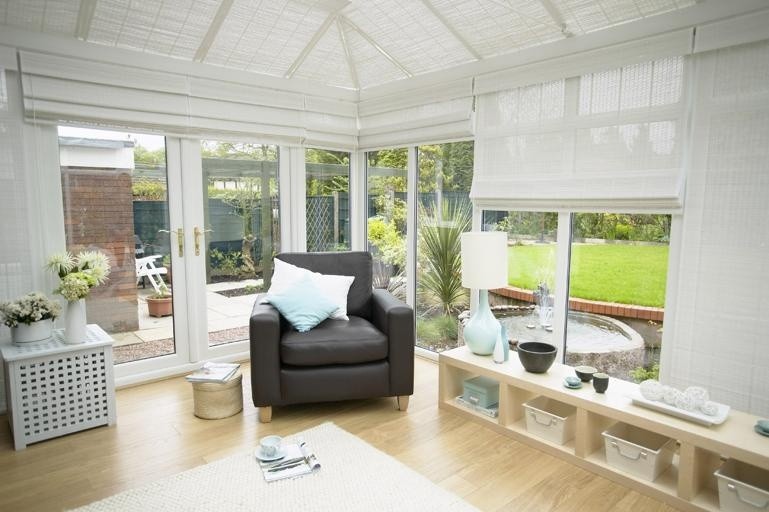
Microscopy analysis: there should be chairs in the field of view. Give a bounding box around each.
[249,250,415,425]
[135,252,168,296]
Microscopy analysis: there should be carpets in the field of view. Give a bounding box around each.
[66,422,484,512]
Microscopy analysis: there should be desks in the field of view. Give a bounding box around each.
[1,325,117,451]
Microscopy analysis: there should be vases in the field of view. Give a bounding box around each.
[10,319,56,346]
[64,298,87,344]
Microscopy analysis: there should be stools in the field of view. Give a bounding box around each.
[192,370,243,421]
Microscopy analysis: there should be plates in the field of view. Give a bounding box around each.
[255,447,287,461]
[564,382,582,388]
[755,426,768,436]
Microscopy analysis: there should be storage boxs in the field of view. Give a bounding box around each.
[601,421,676,482]
[714,457,769,512]
[521,395,577,446]
[462,375,499,408]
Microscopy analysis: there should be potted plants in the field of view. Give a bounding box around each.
[145,286,173,319]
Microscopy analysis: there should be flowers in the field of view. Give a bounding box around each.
[0,292,62,327]
[44,248,112,305]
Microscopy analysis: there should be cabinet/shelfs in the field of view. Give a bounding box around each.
[438,345,769,512]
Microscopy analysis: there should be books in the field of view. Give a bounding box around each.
[259,440,321,482]
[185,364,241,383]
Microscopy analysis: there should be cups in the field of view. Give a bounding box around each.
[260,436,281,457]
[593,373,609,393]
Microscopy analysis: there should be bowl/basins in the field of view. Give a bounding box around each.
[575,366,597,382]
[758,420,769,432]
[566,377,581,385]
[517,342,557,373]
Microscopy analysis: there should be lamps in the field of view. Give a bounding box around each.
[460,231,510,355]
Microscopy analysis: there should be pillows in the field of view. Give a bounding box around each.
[260,258,356,335]
